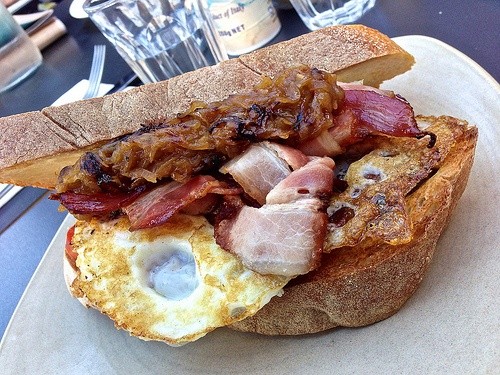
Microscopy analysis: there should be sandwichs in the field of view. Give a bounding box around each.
[0,25,478,347]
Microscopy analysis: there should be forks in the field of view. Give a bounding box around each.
[0,45,106,199]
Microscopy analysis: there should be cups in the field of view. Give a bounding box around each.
[83,0,227,84]
[199,0,281,56]
[289,0,376,33]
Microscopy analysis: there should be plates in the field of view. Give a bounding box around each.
[69,0,89,18]
[0,36,500,375]
